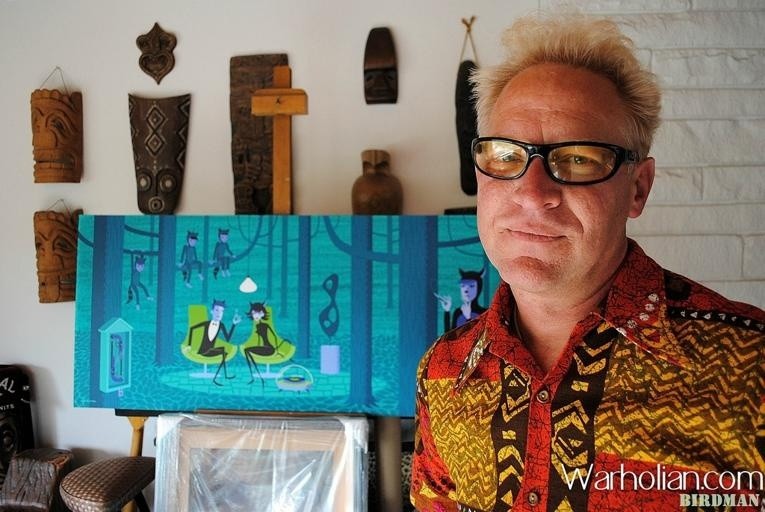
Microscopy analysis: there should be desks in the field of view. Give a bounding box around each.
[168,415,357,510]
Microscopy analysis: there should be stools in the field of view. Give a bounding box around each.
[59,453,157,510]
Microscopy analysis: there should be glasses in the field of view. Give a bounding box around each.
[471,137,640,185]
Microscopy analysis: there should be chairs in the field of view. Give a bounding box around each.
[179,305,294,379]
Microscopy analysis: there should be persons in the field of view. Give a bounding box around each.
[409,22,765,512]
